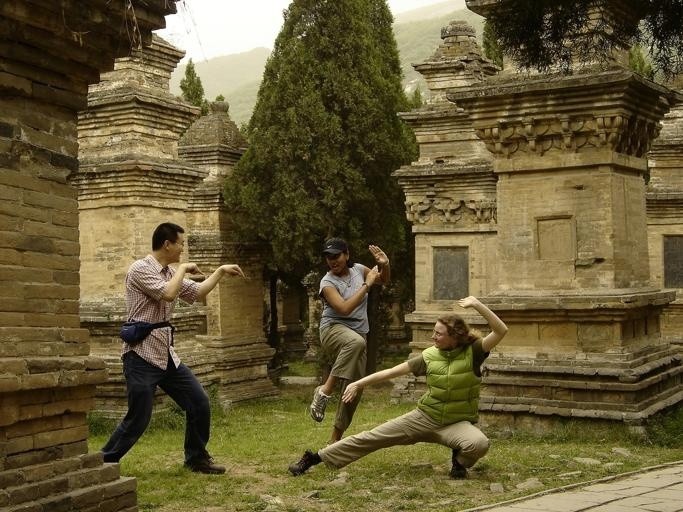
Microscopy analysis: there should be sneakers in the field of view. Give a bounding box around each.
[288,448,316,478]
[449,447,472,481]
[182,455,227,477]
[309,384,333,423]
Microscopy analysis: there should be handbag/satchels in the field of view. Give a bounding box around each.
[117,319,178,348]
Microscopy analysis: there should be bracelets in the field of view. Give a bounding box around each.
[362,283,369,293]
[383,259,389,267]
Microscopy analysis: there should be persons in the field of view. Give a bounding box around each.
[289,296,508,480]
[99,222,247,475]
[309,238,391,446]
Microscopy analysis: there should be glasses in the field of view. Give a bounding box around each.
[432,328,452,338]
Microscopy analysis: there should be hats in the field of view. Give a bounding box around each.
[320,236,349,258]
